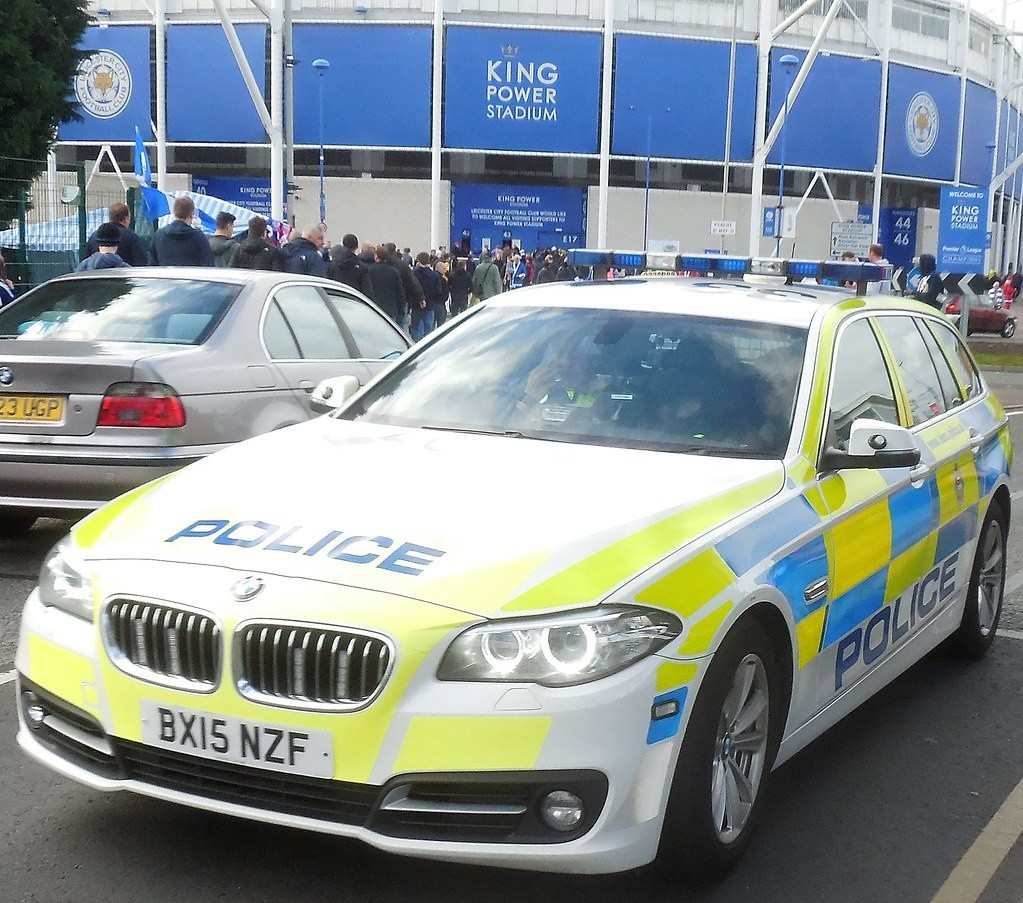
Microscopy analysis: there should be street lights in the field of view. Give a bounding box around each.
[629,105,672,251]
[774,55,800,258]
[985,141,996,188]
[312,59,330,232]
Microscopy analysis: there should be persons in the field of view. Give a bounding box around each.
[208,211,242,268]
[472,251,502,302]
[432,261,449,329]
[465,254,477,277]
[370,245,405,322]
[409,252,442,343]
[147,196,214,266]
[272,224,332,278]
[383,242,426,328]
[0,254,19,309]
[398,245,459,284]
[327,234,373,300]
[823,244,892,295]
[710,346,803,453]
[988,271,1022,310]
[357,242,377,271]
[448,260,474,317]
[478,244,624,290]
[485,319,632,431]
[903,253,944,312]
[82,203,148,267]
[236,215,278,270]
[76,222,131,273]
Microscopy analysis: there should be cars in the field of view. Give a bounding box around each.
[945,294,1018,339]
[0,265,417,536]
[13,248,1014,877]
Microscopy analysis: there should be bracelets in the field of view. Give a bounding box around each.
[519,392,537,408]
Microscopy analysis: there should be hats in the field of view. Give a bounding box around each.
[96,223,122,246]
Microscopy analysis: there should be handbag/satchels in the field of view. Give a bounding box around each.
[472,284,484,296]
